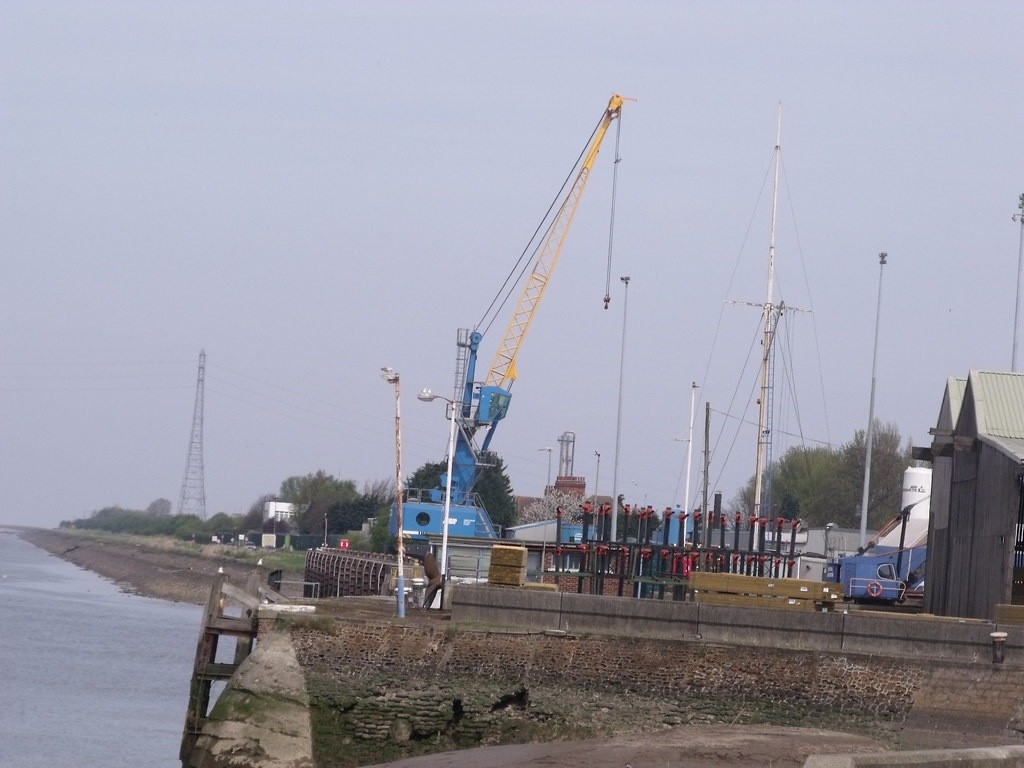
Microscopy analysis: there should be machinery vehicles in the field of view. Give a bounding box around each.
[387,90,638,562]
[823,495,931,608]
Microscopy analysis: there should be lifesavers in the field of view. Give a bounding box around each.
[867,581,883,597]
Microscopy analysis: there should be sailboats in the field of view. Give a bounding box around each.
[682,100,826,576]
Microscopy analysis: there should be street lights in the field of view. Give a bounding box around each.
[380,367,405,618]
[610,276,632,542]
[1011,193,1024,372]
[418,390,457,609]
[537,448,553,583]
[858,252,888,549]
[683,382,701,546]
